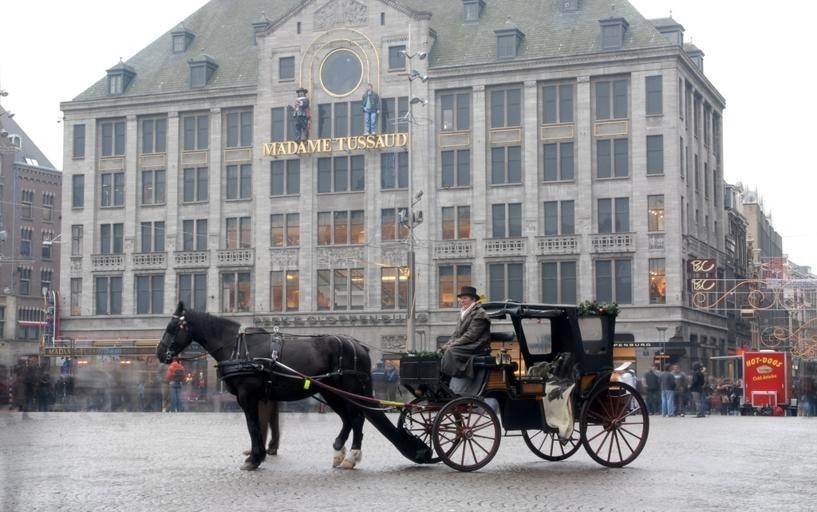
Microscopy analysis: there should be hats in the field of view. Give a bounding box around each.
[457,286,480,300]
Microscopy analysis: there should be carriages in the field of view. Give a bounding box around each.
[156,300,649,471]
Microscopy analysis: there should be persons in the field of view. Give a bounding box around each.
[0,358,186,415]
[185,372,193,400]
[291,86,311,143]
[360,82,382,137]
[608,360,816,419]
[195,372,205,400]
[435,285,493,399]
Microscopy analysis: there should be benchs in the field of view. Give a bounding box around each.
[550,351,578,379]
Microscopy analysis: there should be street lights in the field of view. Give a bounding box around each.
[394,49,430,356]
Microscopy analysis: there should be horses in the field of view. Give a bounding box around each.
[154,299,374,474]
